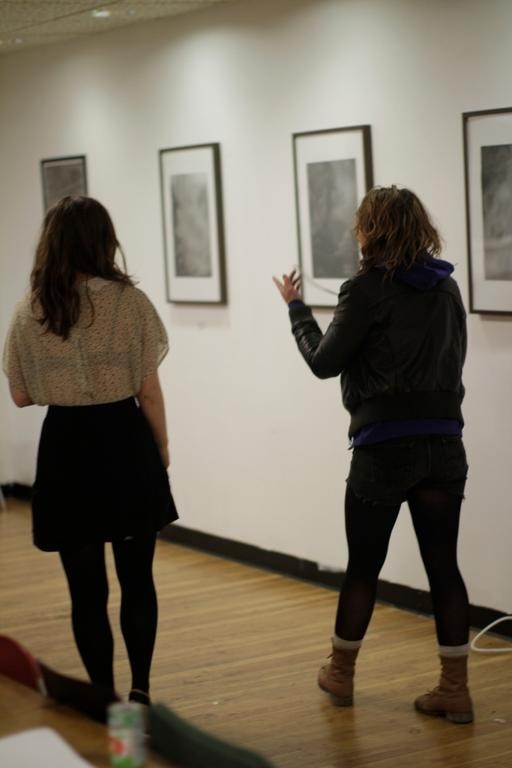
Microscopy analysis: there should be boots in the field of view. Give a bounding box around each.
[317,647,360,705]
[416,655,473,724]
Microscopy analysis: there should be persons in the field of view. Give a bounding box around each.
[270,185,474,726]
[1,194,180,743]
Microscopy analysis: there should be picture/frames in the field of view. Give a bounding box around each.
[463,110,511,313]
[156,140,229,306]
[39,153,88,222]
[293,125,372,309]
